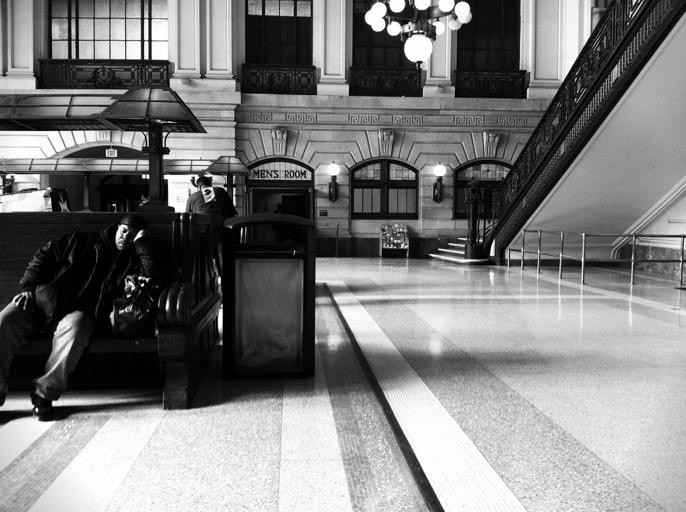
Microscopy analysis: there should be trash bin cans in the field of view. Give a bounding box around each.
[221,213,316,380]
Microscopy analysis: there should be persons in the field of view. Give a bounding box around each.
[0,214,159,416]
[187,176,239,277]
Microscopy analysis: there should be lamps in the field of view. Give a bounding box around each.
[360,1,473,70]
[431,161,448,204]
[325,159,342,202]
[206,154,252,206]
[94,80,205,213]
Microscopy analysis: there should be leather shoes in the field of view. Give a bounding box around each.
[28,392,54,418]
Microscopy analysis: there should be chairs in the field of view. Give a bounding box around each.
[377,222,412,259]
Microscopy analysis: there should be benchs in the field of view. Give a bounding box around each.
[0,211,225,414]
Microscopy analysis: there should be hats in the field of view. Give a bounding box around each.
[120,213,149,232]
